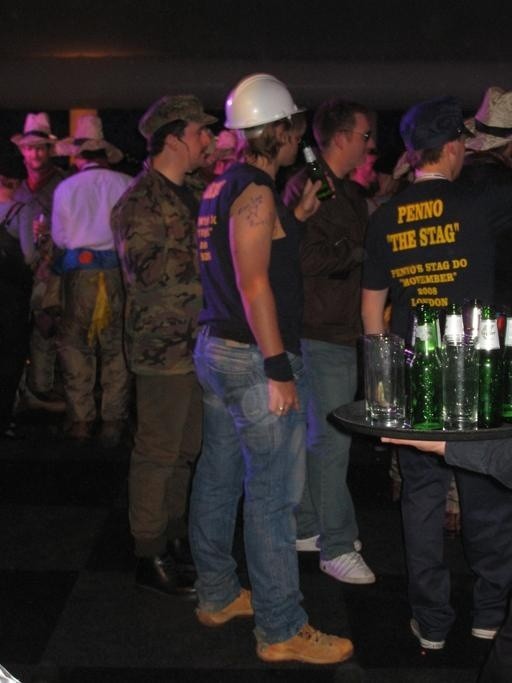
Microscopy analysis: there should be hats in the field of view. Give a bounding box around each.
[462,85,512,154]
[9,111,58,146]
[137,93,221,141]
[55,113,125,165]
[399,99,474,152]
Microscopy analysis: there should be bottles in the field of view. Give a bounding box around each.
[410,297,511,431]
[298,138,334,201]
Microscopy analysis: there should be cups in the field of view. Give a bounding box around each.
[364,332,406,424]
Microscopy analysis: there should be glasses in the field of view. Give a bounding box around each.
[338,130,373,142]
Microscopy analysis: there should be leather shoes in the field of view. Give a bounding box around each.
[163,537,197,563]
[133,555,200,598]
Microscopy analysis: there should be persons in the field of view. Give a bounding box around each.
[1,72,512,664]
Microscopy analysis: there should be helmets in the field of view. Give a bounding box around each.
[222,71,308,132]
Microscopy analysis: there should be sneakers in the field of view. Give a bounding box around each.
[196,587,254,627]
[97,420,126,449]
[408,616,445,651]
[317,550,375,584]
[2,412,33,441]
[65,420,92,444]
[254,623,354,664]
[471,622,500,642]
[295,534,362,552]
